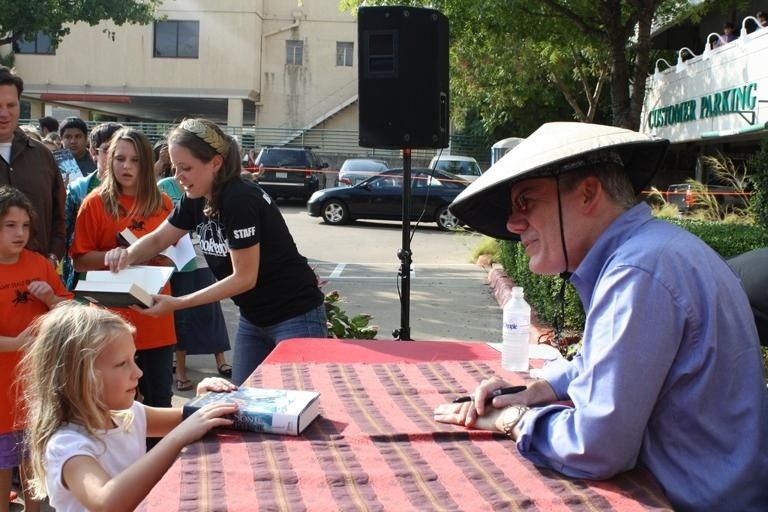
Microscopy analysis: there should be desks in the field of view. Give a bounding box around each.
[132,335,676,512]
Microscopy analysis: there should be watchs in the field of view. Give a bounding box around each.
[500,403,531,441]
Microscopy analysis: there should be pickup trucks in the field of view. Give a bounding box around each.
[667,176,756,218]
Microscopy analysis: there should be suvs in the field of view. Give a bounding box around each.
[253,142,330,206]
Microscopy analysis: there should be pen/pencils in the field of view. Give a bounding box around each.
[453,386,527,403]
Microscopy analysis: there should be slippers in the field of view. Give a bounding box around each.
[175,380,192,391]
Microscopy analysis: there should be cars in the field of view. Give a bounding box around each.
[306,166,474,232]
[424,155,482,188]
[334,157,400,189]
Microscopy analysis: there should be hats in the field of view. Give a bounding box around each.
[449,122,670,240]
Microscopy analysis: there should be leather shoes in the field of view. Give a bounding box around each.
[217,364,232,376]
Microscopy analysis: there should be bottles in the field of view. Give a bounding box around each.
[501,284,532,374]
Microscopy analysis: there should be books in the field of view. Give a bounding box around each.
[183,388,320,436]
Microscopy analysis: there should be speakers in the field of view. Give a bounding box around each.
[356,6,450,151]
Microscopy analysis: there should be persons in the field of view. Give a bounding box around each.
[18,116,123,293]
[434,121,767,510]
[1,188,76,511]
[0,63,69,486]
[65,126,182,456]
[153,141,233,390]
[16,300,236,511]
[105,118,329,385]
